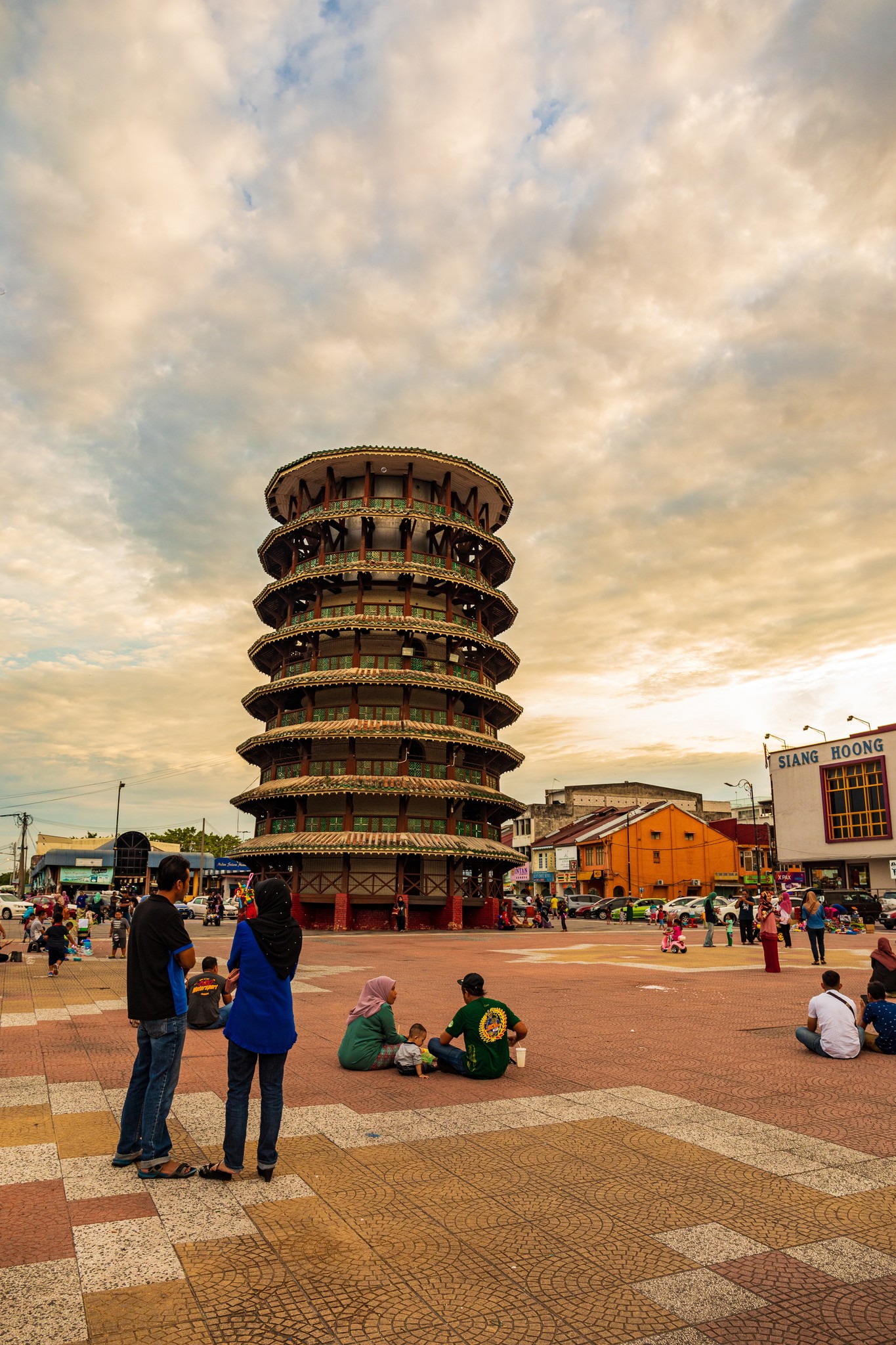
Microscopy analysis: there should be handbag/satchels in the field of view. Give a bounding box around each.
[502,919,506,924]
[550,906,551,910]
[805,925,807,930]
[6,950,22,962]
[564,915,567,919]
[714,914,719,924]
[788,918,793,925]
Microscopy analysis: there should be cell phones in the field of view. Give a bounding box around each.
[9,939,15,942]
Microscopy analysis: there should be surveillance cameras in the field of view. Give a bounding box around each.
[407,752,410,754]
[455,756,456,757]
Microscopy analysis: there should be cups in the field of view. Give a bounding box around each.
[536,925,539,928]
[516,1048,526,1067]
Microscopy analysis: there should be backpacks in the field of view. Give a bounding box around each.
[59,903,70,921]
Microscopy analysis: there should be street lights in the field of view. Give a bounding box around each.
[724,779,763,894]
[237,831,251,843]
[626,809,646,897]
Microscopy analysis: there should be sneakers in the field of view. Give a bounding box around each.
[48,963,59,977]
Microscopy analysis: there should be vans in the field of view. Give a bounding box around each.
[73,890,123,905]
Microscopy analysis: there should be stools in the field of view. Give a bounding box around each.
[19,918,26,924]
[27,941,40,953]
[688,918,698,928]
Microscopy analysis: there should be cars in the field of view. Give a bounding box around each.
[84,894,121,919]
[25,894,78,920]
[231,894,239,909]
[139,895,191,919]
[187,896,238,920]
[224,898,233,904]
[504,889,896,931]
[0,893,37,920]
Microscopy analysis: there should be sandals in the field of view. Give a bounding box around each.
[811,961,820,965]
[110,1147,197,1178]
[821,960,826,964]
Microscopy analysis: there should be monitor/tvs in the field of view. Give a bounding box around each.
[449,653,460,662]
[402,647,415,656]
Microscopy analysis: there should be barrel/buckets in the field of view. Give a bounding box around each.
[77,931,89,946]
[667,921,672,927]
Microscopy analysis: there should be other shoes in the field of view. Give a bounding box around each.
[743,942,759,945]
[120,955,126,959]
[655,924,657,925]
[625,923,627,924]
[703,945,716,947]
[561,930,568,932]
[725,945,732,947]
[43,949,48,953]
[557,916,559,920]
[551,918,554,920]
[630,923,632,924]
[782,945,793,949]
[108,955,115,959]
[437,1057,449,1073]
[647,924,650,925]
[607,923,611,924]
[22,941,26,942]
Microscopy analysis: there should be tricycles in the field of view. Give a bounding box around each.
[660,929,687,953]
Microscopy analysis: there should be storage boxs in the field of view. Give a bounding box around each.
[790,920,799,929]
[677,935,686,941]
[864,924,875,934]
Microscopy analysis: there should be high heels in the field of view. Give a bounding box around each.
[255,1165,273,1183]
[199,1162,231,1181]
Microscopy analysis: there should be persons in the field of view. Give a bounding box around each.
[727,894,735,902]
[112,854,199,1180]
[428,973,528,1080]
[719,912,734,947]
[0,886,158,961]
[703,892,719,947]
[734,889,760,945]
[868,937,896,998]
[44,913,78,977]
[337,976,410,1071]
[862,887,866,892]
[499,892,570,932]
[393,896,407,932]
[801,891,826,965]
[664,918,683,948]
[758,891,785,973]
[794,970,865,1059]
[187,956,234,1030]
[181,967,191,1012]
[394,1023,437,1079]
[775,892,793,948]
[825,903,848,920]
[189,897,196,902]
[204,891,225,925]
[604,889,685,930]
[199,877,303,1182]
[851,887,855,891]
[856,981,896,1054]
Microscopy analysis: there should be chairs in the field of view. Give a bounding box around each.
[852,895,857,900]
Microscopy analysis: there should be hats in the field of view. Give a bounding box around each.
[457,972,485,989]
[217,894,220,897]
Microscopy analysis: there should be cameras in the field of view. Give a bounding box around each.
[742,895,744,898]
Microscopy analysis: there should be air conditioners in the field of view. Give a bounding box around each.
[657,879,663,885]
[692,879,700,886]
[764,871,771,875]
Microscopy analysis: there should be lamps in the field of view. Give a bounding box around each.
[847,715,871,731]
[765,734,786,750]
[449,654,459,664]
[803,725,827,742]
[402,647,414,657]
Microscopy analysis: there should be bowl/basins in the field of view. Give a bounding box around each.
[73,958,82,961]
